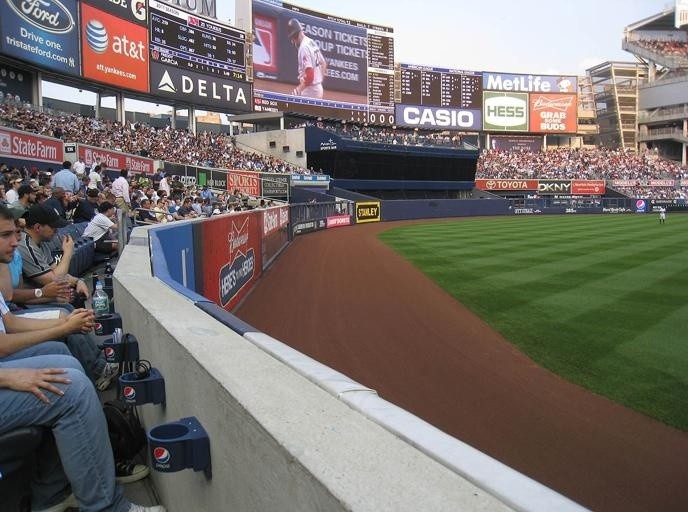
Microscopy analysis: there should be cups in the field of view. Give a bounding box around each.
[52,274,72,305]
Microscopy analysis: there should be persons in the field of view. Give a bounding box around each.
[659,205,667,224]
[1,202,170,512]
[0,159,281,224]
[632,39,687,60]
[477,142,687,206]
[0,93,324,175]
[285,18,330,98]
[326,120,464,148]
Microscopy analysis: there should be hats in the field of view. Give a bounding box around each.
[17,184,38,197]
[7,175,23,185]
[25,203,71,229]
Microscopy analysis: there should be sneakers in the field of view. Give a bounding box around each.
[127,502,167,512]
[115,462,151,484]
[94,362,120,392]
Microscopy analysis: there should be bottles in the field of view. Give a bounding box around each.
[112,327,123,344]
[104,264,113,287]
[91,281,110,320]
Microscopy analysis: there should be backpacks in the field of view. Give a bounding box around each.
[103,398,149,460]
